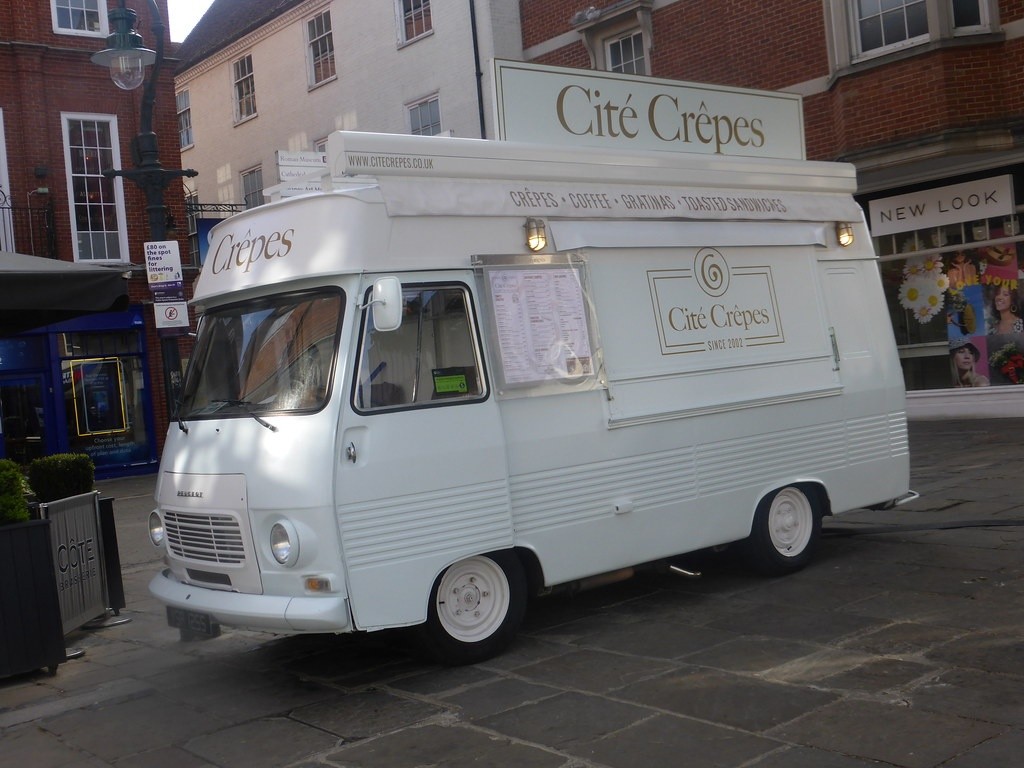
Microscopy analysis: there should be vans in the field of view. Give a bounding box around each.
[146,127,924,662]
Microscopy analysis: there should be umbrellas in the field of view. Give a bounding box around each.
[0,251,144,339]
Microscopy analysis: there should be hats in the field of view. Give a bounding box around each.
[947,338,980,362]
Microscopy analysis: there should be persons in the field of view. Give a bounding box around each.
[949,337,990,387]
[987,283,1024,334]
[946,249,976,288]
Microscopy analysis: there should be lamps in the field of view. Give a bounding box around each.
[165,205,178,238]
[523,217,548,252]
[835,222,855,246]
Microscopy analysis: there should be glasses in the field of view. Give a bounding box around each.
[952,252,966,257]
